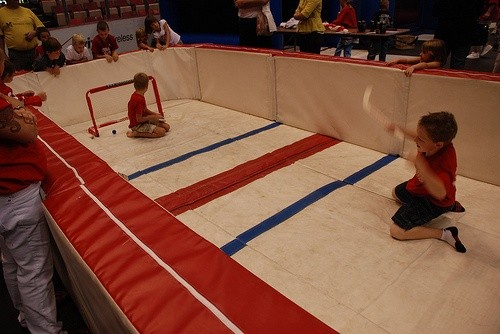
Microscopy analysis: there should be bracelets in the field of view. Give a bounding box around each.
[13,105,22,110]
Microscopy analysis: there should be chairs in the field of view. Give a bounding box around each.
[39,0,159,31]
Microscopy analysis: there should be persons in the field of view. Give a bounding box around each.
[34,26,51,61]
[294,0,323,55]
[366,0,393,64]
[386,108,468,252]
[235,0,277,49]
[92,20,119,62]
[66,32,91,65]
[127,72,170,137]
[480,0,500,21]
[431,0,484,70]
[388,38,449,78]
[0,0,45,72]
[333,0,358,59]
[136,28,154,52]
[0,47,74,334]
[32,37,66,77]
[145,16,184,49]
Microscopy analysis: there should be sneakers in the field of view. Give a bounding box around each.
[480,45,493,56]
[467,51,480,59]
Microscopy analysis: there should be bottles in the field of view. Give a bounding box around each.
[357,21,361,32]
[377,21,386,34]
[362,21,365,32]
[370,21,375,32]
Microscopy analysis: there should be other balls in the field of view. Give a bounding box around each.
[112,129,116,134]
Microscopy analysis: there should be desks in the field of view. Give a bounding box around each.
[272,25,410,52]
